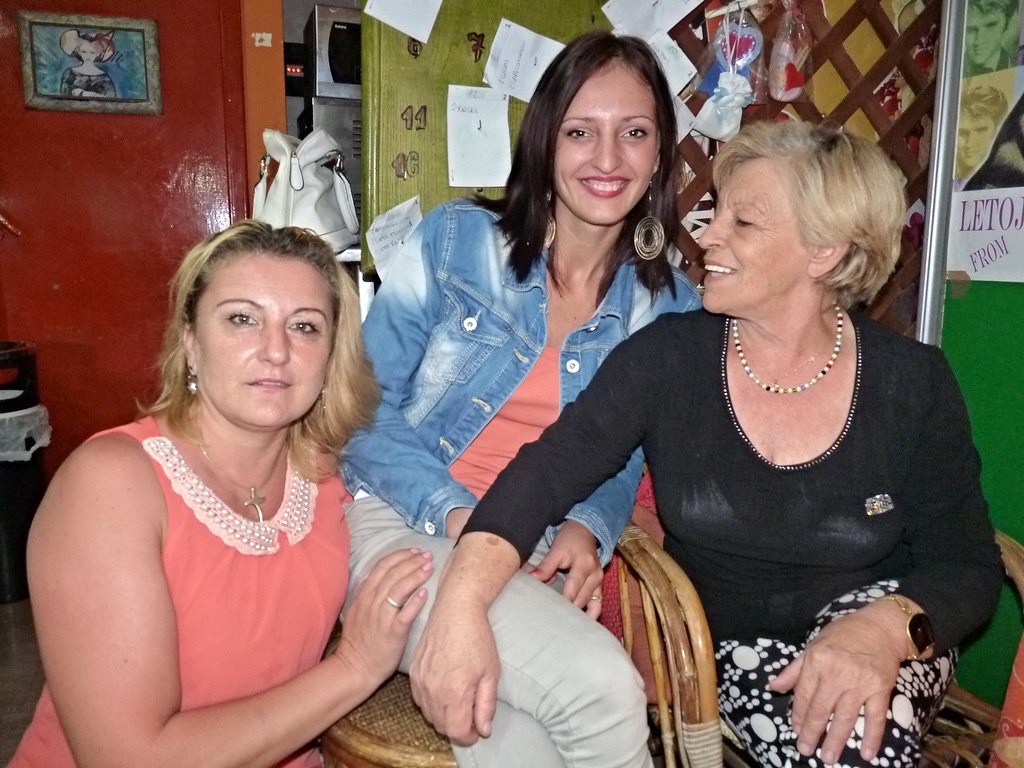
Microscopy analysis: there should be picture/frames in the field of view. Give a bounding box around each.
[18,10,163,116]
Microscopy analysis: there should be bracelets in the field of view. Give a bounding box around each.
[883,593,935,661]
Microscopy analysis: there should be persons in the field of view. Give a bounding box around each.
[409,120,1006,768]
[955,86,1008,178]
[334,28,704,768]
[9,218,434,768]
[963,0,1019,78]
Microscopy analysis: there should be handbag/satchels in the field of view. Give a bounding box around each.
[250,126,360,255]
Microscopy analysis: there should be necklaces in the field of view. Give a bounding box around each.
[732,302,843,394]
[200,446,266,523]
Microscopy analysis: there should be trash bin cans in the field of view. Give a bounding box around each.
[0,340,47,605]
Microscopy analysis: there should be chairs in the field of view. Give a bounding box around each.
[324,462,1024,767]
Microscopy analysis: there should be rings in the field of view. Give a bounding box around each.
[386,597,402,608]
[591,596,601,601]
